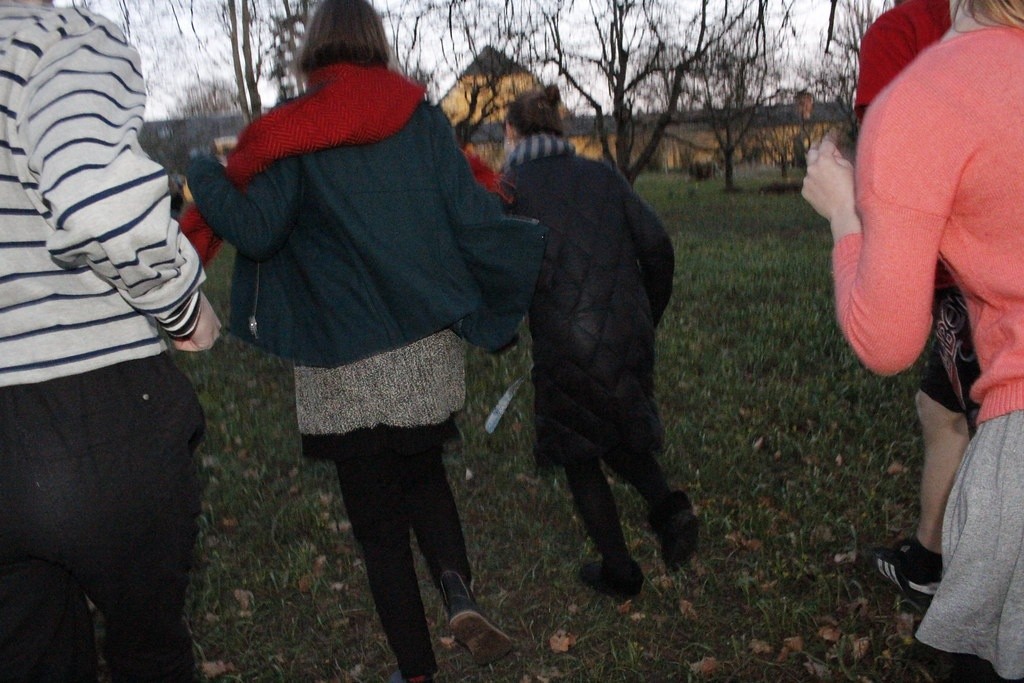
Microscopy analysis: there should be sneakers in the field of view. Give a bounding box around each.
[870,535,942,602]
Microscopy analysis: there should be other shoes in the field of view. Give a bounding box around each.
[390,667,433,683]
[440,570,514,665]
[648,491,701,566]
[582,558,643,599]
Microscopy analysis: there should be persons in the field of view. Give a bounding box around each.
[3,1,223,682]
[173,0,548,682]
[797,2,1019,683]
[489,82,703,600]
